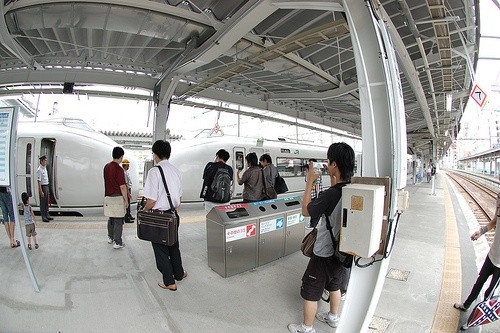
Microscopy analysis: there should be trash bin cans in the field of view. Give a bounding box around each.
[206,196,305,278]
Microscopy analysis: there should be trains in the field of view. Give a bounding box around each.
[168,135,423,203]
[16,116,139,217]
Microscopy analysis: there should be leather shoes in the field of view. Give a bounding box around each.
[177,270,188,281]
[158,281,177,291]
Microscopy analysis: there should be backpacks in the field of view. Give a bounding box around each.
[208,162,233,203]
[323,185,353,268]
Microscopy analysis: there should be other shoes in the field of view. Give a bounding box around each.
[130,216,135,220]
[460,322,469,330]
[34,244,39,249]
[454,303,467,311]
[43,219,49,222]
[10,240,21,248]
[47,218,53,220]
[28,244,32,249]
[125,220,134,223]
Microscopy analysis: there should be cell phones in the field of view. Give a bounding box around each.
[312,162,328,168]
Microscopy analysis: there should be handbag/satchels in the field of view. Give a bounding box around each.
[103,196,127,218]
[137,208,178,246]
[301,228,318,257]
[274,173,288,194]
[467,277,500,327]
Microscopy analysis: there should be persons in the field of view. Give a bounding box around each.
[426,164,436,182]
[260,153,276,199]
[0,186,20,249]
[304,159,322,197]
[121,159,135,223]
[237,153,264,203]
[454,194,500,331]
[20,192,39,250]
[203,149,233,212]
[103,146,128,249]
[143,141,187,291]
[37,154,54,223]
[287,141,355,333]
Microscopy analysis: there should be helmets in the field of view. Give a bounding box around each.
[122,159,130,164]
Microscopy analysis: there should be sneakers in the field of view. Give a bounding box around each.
[315,311,339,328]
[287,322,317,333]
[112,242,126,249]
[107,236,113,244]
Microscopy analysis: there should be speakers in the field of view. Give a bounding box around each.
[63,83,74,93]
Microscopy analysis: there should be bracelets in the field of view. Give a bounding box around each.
[482,227,488,234]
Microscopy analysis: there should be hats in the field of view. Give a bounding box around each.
[39,154,48,160]
